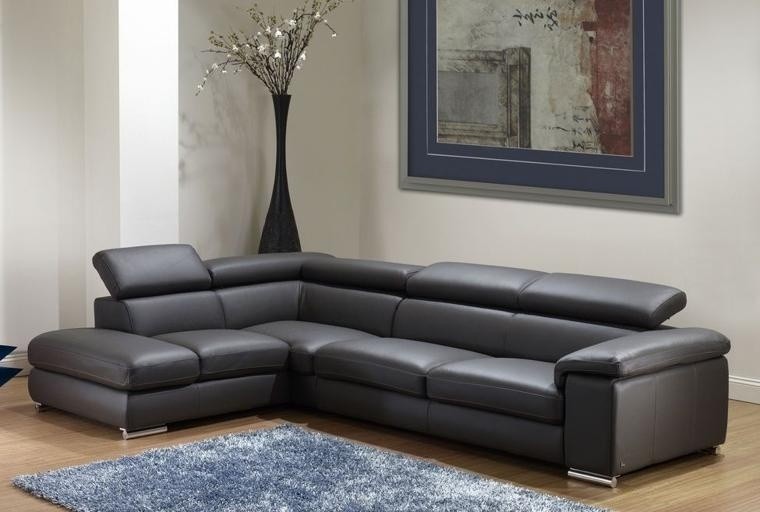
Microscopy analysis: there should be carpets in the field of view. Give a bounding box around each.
[9,422,616,512]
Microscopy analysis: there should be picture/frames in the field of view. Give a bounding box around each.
[399,1,683,217]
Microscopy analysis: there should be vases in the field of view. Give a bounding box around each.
[258,94,302,254]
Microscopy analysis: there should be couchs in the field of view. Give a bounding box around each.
[27,244,731,488]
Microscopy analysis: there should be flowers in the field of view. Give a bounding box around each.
[195,0,350,97]
